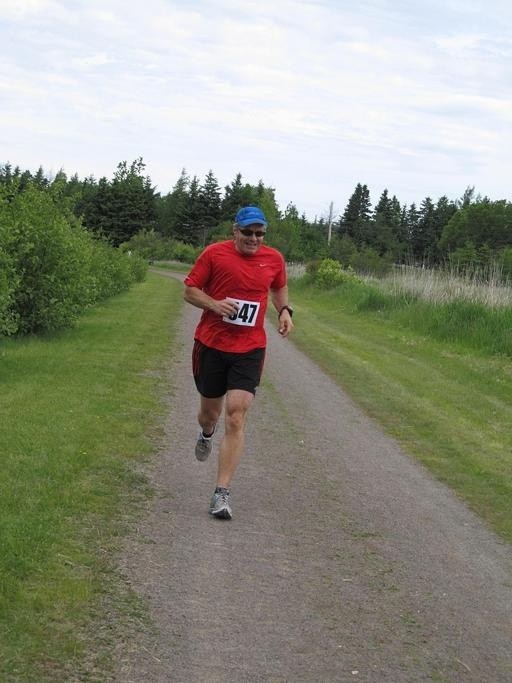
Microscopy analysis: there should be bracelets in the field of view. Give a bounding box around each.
[277,303,294,319]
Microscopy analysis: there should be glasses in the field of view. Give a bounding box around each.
[239,228,267,236]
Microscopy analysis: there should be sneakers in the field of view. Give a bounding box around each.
[195,425,216,460]
[210,487,232,520]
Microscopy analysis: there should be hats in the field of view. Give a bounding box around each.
[235,208,267,227]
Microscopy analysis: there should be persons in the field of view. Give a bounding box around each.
[182,206,294,521]
